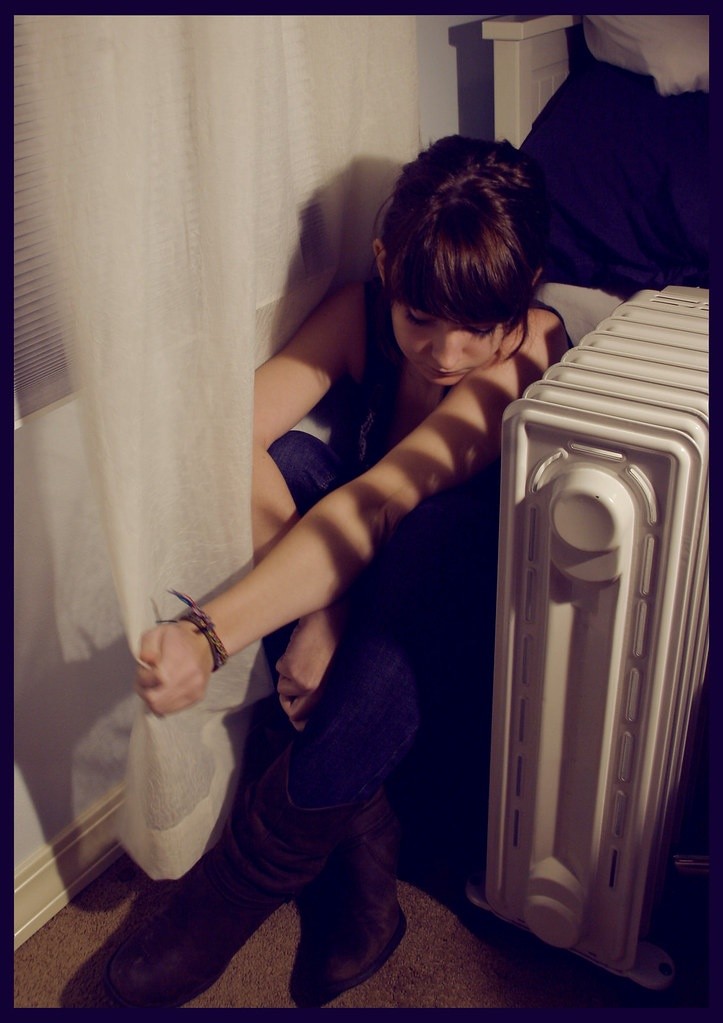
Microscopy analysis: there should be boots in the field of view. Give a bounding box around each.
[102,739,369,1010]
[286,784,409,1010]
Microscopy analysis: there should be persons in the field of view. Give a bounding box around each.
[103,134,575,1008]
[519,16,710,346]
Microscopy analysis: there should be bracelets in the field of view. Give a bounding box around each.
[165,589,229,674]
